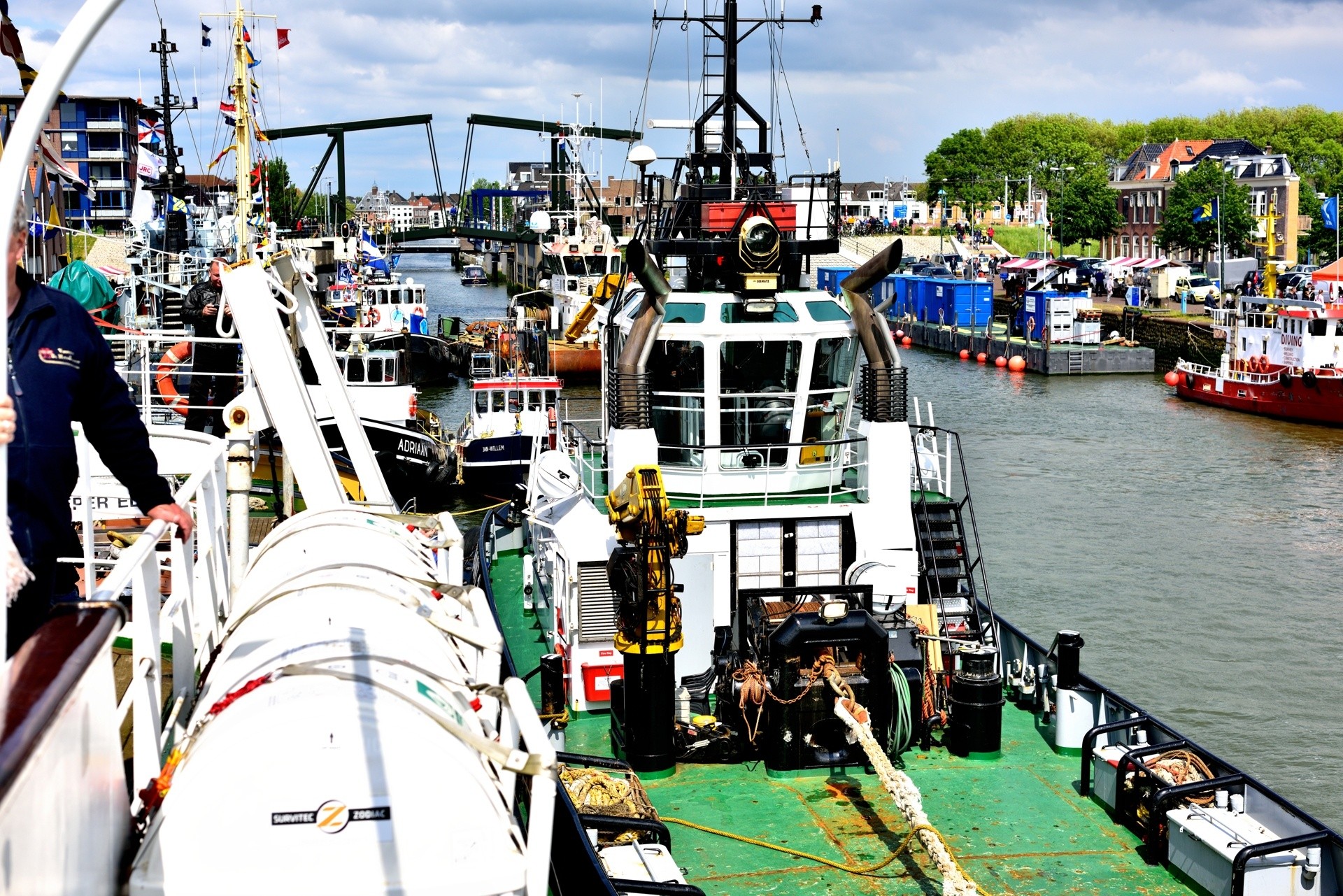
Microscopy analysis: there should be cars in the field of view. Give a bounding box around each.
[1174,260,1220,304]
[1024,251,1108,285]
[1258,265,1338,303]
[898,255,958,280]
[968,255,1021,273]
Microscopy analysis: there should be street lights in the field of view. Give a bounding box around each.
[311,164,320,215]
[940,177,948,253]
[484,207,493,230]
[286,181,292,219]
[1050,166,1075,259]
[1209,156,1239,293]
[320,177,333,222]
[324,181,336,234]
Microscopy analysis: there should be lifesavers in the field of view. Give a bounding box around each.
[410,397,418,416]
[367,308,381,323]
[155,342,217,416]
[548,408,556,450]
[1259,356,1269,373]
[1027,317,1035,331]
[509,399,521,410]
[1248,355,1259,373]
[412,307,424,318]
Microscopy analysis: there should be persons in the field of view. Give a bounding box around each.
[1095,270,1152,302]
[350,217,358,235]
[918,254,931,263]
[524,218,530,226]
[839,215,915,236]
[450,204,470,227]
[0,203,194,662]
[1204,281,1343,327]
[180,258,238,439]
[949,218,1011,282]
[297,216,319,236]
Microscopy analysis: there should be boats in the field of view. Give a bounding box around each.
[1165,296,1343,424]
[0,0,1343,896]
[95,0,564,486]
[506,76,633,371]
[458,264,488,286]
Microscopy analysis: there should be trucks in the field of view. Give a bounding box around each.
[1206,257,1258,294]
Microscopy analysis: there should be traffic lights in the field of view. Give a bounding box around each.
[451,226,457,236]
[484,239,491,251]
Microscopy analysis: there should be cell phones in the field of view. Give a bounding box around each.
[211,305,226,314]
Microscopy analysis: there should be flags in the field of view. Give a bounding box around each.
[165,193,191,216]
[26,196,64,242]
[1191,198,1218,224]
[209,23,269,230]
[1321,196,1339,231]
[336,260,354,284]
[137,145,167,180]
[276,28,291,50]
[360,226,392,274]
[127,176,156,230]
[137,118,165,143]
[38,128,96,202]
[201,22,212,47]
[0,16,69,104]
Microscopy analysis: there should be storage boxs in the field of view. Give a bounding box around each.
[905,278,925,320]
[926,277,995,327]
[871,272,930,317]
[816,267,858,297]
[1024,289,1102,345]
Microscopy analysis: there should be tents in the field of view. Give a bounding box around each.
[1312,255,1343,302]
[1102,254,1192,298]
[997,257,1078,274]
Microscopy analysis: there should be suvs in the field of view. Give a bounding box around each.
[932,254,964,275]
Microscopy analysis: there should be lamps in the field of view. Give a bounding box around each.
[569,243,578,252]
[563,229,569,237]
[594,245,603,252]
[733,213,781,272]
[528,362,535,376]
[741,294,779,317]
